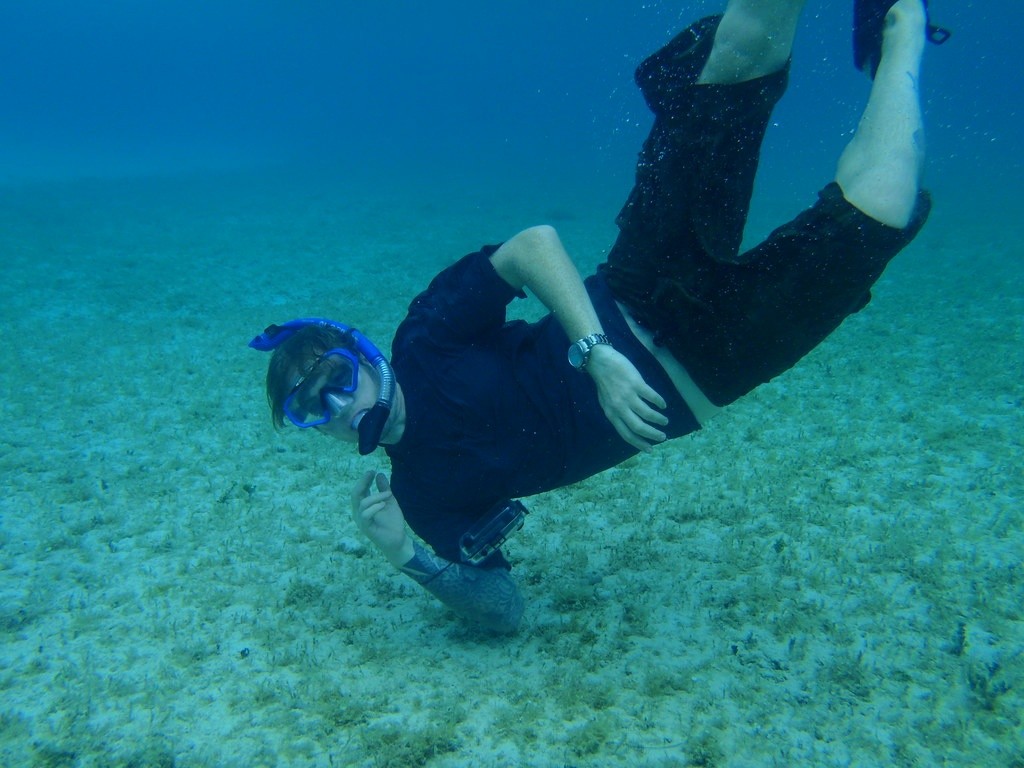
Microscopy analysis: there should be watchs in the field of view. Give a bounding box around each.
[568,334,612,370]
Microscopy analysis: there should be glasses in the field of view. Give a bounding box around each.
[282,348,358,428]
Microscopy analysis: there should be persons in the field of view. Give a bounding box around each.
[267,0,949,635]
